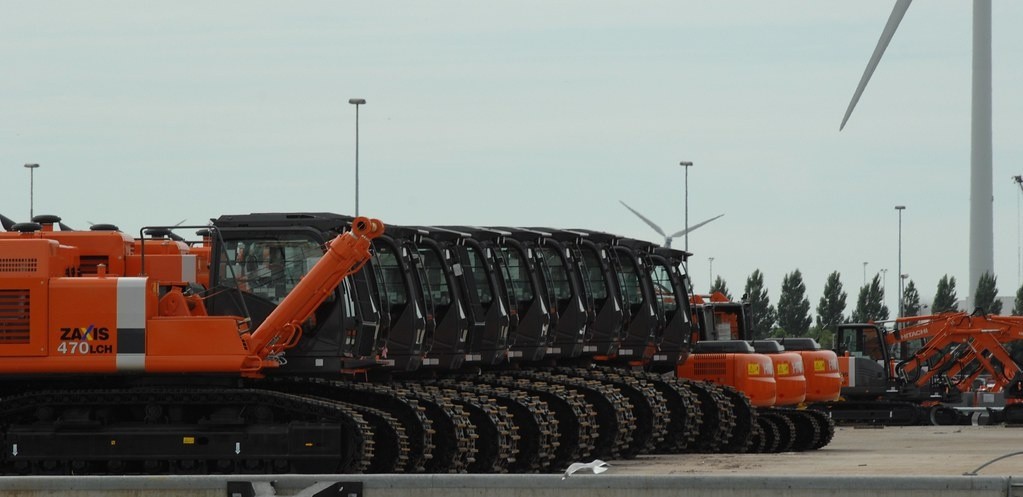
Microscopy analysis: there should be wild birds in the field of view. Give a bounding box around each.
[561,459,612,480]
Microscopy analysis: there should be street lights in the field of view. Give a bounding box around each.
[23,163,40,222]
[879,267,889,306]
[707,257,716,289]
[893,206,907,331]
[678,161,692,293]
[862,261,869,287]
[348,97,366,217]
[901,274,909,329]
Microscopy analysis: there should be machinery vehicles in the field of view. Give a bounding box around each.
[0,209,1023,477]
